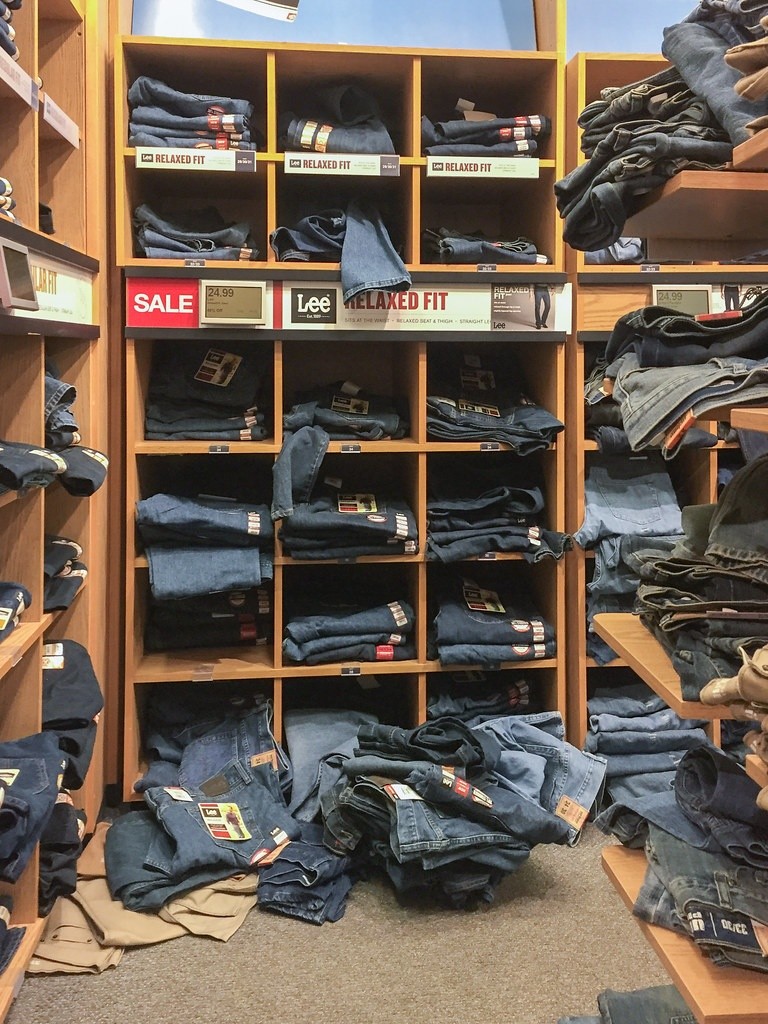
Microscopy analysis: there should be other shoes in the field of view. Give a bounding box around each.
[242,835,245,838]
[536,323,541,329]
[239,836,242,838]
[542,322,548,327]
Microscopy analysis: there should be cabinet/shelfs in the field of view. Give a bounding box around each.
[563,52,768,748]
[112,32,567,803]
[593,170,768,1024]
[0,0,109,1024]
